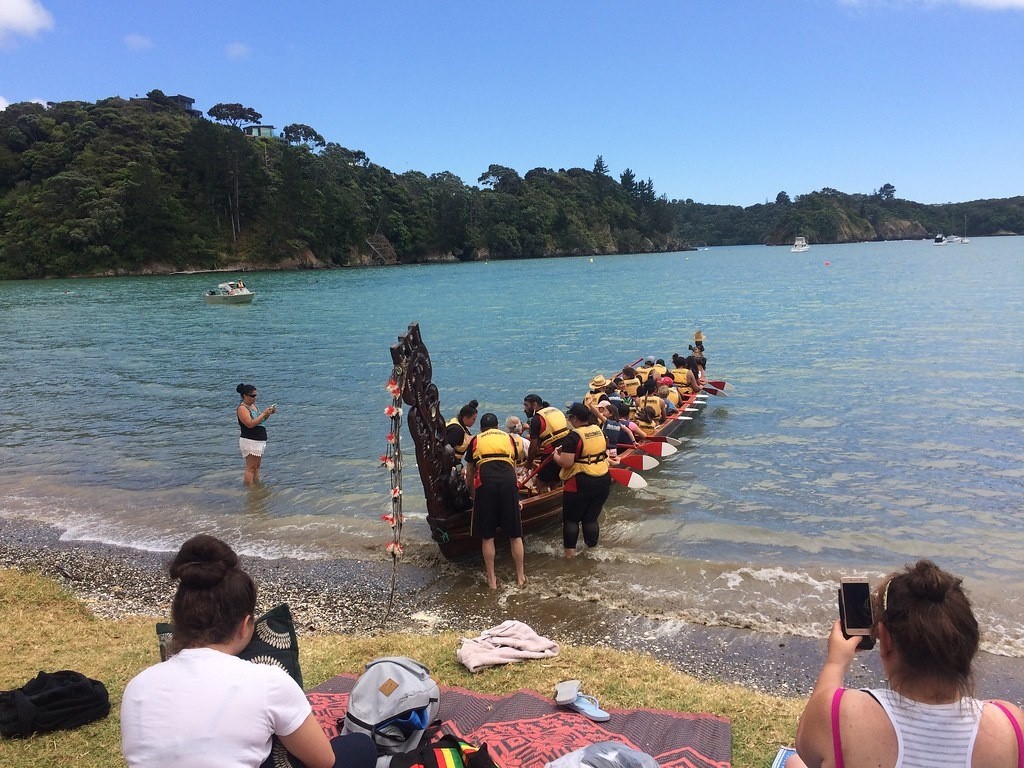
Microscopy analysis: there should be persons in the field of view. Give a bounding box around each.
[236,383,277,481]
[583,353,707,438]
[463,414,526,589]
[605,438,621,465]
[228,285,235,294]
[553,403,611,558]
[585,394,640,458]
[237,279,245,288]
[688,330,706,371]
[445,393,570,495]
[121,533,378,768]
[784,558,1024,768]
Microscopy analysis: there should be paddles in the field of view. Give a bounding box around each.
[518,445,563,488]
[635,435,683,446]
[611,454,662,471]
[610,357,644,380]
[678,407,699,412]
[607,466,649,489]
[666,415,693,420]
[617,440,679,457]
[703,387,729,398]
[683,393,709,397]
[701,380,736,390]
[682,400,706,404]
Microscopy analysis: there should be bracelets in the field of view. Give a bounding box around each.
[632,440,635,443]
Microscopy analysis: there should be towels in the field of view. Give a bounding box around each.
[457,619,560,674]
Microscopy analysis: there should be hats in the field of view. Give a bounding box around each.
[607,382,617,391]
[566,402,582,410]
[661,373,674,380]
[657,376,674,387]
[594,400,611,408]
[647,356,655,364]
[589,375,611,389]
[621,364,631,374]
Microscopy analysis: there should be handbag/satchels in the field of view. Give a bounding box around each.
[544,741,660,768]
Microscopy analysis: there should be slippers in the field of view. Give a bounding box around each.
[564,692,611,722]
[555,680,582,705]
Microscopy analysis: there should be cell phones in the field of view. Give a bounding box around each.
[841,576,874,635]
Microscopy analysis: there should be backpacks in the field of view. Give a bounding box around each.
[389,733,500,768]
[0,670,111,740]
[338,655,441,768]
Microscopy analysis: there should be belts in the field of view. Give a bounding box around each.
[475,454,509,464]
[540,428,567,442]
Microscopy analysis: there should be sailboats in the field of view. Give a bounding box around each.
[951,208,970,244]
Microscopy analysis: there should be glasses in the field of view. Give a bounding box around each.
[244,393,256,398]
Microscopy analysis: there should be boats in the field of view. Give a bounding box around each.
[704,248,708,250]
[946,234,959,242]
[390,321,708,559]
[204,282,255,304]
[932,233,948,246]
[790,237,810,252]
